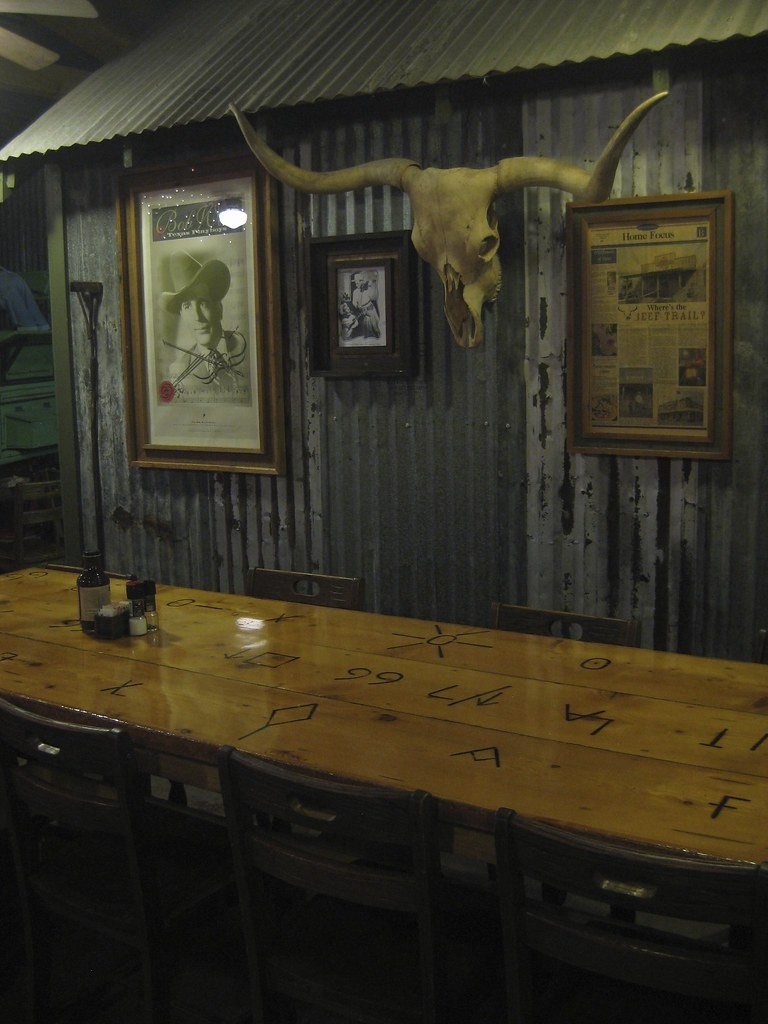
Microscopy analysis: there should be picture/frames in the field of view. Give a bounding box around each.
[111,153,287,477]
[306,230,418,379]
[565,189,734,463]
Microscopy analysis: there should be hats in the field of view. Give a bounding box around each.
[158,250,231,315]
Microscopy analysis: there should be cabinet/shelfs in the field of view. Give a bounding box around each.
[0,296,59,465]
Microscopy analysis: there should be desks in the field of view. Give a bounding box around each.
[0,567,768,872]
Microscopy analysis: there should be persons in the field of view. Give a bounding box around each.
[161,249,250,394]
[339,274,382,340]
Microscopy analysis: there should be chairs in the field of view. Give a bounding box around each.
[0,698,234,1024]
[0,479,64,570]
[249,564,365,611]
[217,744,504,1024]
[494,807,768,1024]
[490,601,641,648]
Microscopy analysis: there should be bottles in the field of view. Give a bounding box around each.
[129,615,147,636]
[76,551,111,632]
[142,580,156,612]
[144,610,159,631]
[126,574,145,618]
